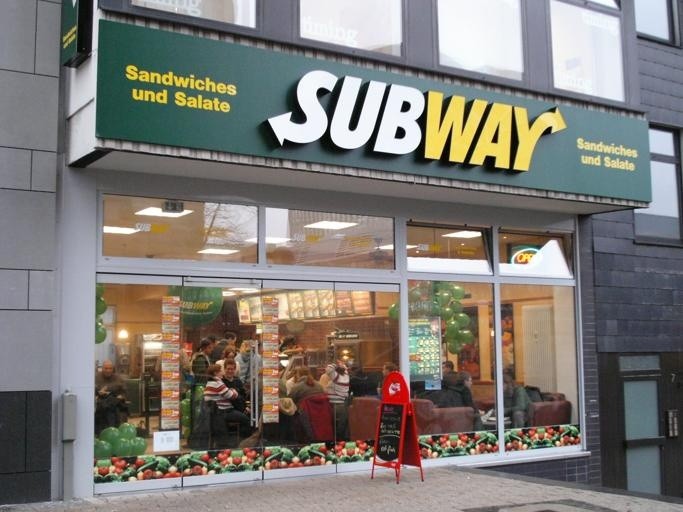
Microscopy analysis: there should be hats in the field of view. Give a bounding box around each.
[278,397,297,416]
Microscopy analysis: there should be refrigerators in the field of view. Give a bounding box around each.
[133,332,163,415]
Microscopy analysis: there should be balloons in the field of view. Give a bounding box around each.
[180,385,204,435]
[94,283,107,344]
[94,423,147,463]
[389,284,473,353]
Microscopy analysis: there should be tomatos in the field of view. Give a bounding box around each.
[94,423,582,483]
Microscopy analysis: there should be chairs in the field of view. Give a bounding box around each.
[200,397,241,449]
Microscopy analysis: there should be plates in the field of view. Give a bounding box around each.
[485,416,509,421]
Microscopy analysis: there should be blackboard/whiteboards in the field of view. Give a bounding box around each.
[374,402,407,468]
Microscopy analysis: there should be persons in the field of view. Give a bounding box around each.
[189,325,369,447]
[502,370,532,428]
[415,360,454,402]
[375,362,398,399]
[440,371,485,431]
[94,360,131,433]
